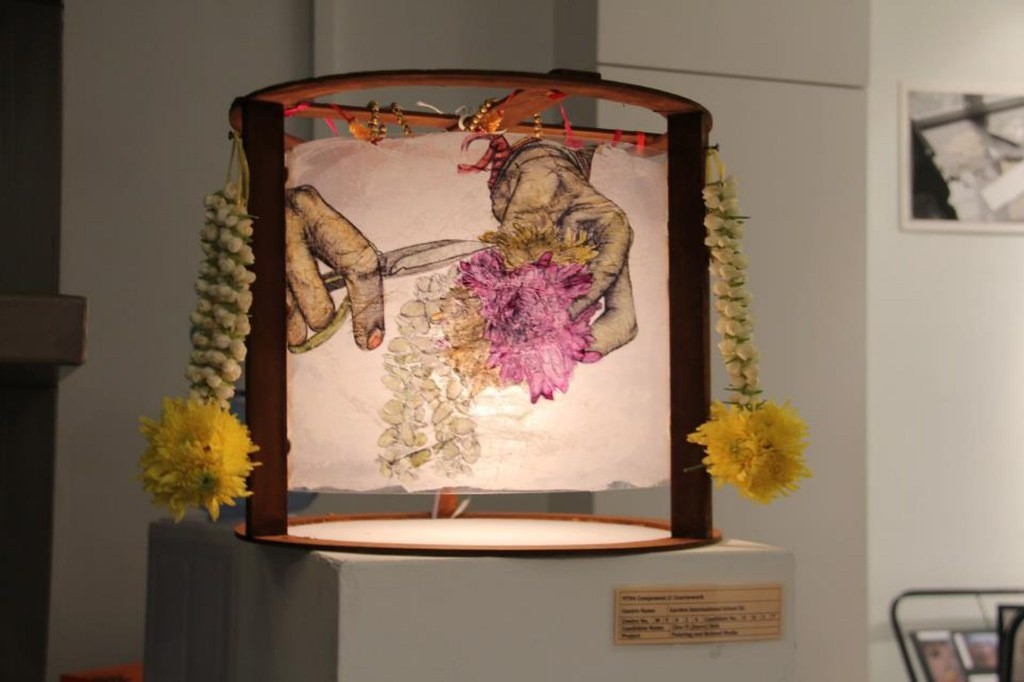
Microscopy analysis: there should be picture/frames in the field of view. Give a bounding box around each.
[899,77,1024,233]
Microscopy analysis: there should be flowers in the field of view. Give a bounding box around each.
[685,150,816,502]
[137,183,259,525]
